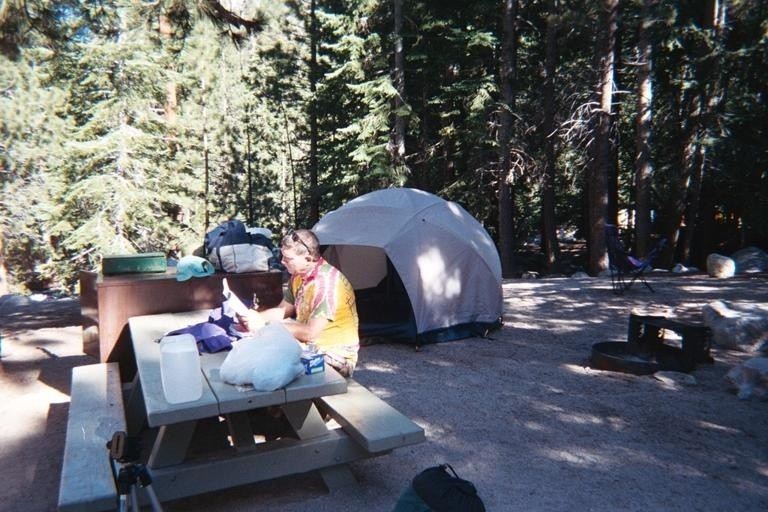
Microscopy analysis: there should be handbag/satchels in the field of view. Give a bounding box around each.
[201,220,277,274]
[390,463,485,512]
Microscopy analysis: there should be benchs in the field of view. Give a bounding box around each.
[57,361,128,512]
[312,377,425,462]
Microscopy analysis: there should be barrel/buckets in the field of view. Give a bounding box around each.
[159,330,203,405]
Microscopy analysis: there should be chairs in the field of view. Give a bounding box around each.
[603,223,667,297]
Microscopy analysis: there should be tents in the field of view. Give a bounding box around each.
[311,186,504,346]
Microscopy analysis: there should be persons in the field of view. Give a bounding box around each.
[236,230,360,440]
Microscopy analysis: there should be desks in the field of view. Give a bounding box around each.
[78,269,284,384]
[122,307,358,511]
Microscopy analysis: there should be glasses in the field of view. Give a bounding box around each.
[287,228,311,256]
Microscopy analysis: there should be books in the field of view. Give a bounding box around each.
[221,277,248,318]
